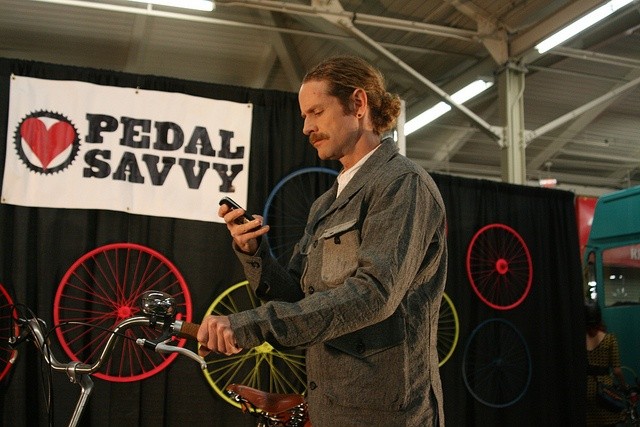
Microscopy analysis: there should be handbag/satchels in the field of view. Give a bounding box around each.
[597,381,627,412]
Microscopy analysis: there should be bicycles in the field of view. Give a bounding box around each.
[7,289,309,425]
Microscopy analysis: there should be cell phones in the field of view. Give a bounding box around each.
[219,196,264,243]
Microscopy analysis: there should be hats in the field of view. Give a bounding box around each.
[587,302,601,324]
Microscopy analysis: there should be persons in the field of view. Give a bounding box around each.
[586,301,638,427]
[196,54,450,426]
